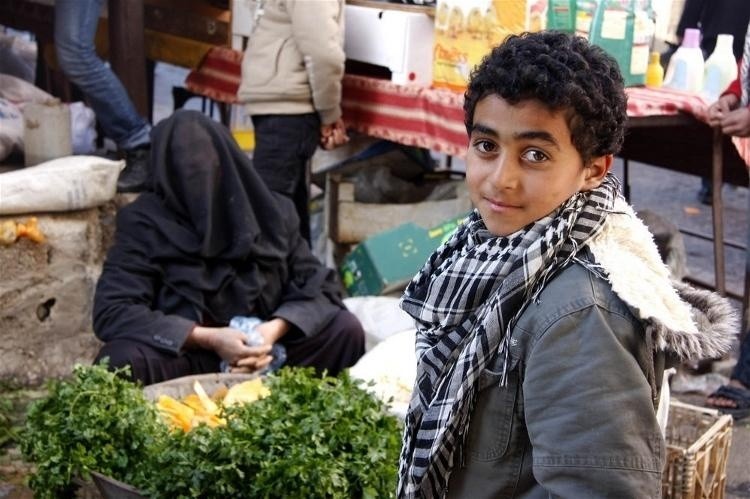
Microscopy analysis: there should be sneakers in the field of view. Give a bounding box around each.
[114,141,154,193]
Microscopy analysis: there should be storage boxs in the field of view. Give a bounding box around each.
[323,173,475,246]
[336,212,478,296]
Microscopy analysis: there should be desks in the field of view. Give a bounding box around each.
[180,45,750,302]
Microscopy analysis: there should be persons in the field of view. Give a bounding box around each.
[398,30,740,499]
[236,0,349,252]
[92,112,364,387]
[660,0,750,212]
[55,1,154,153]
[706,22,750,421]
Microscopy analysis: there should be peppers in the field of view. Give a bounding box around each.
[157,392,227,433]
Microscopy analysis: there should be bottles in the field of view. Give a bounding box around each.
[663,27,704,100]
[645,50,665,88]
[701,32,738,102]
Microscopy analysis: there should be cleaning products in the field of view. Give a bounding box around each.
[644,27,738,101]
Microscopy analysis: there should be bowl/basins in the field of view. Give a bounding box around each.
[85,372,267,499]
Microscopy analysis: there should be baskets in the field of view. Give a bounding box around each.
[667,398,734,498]
